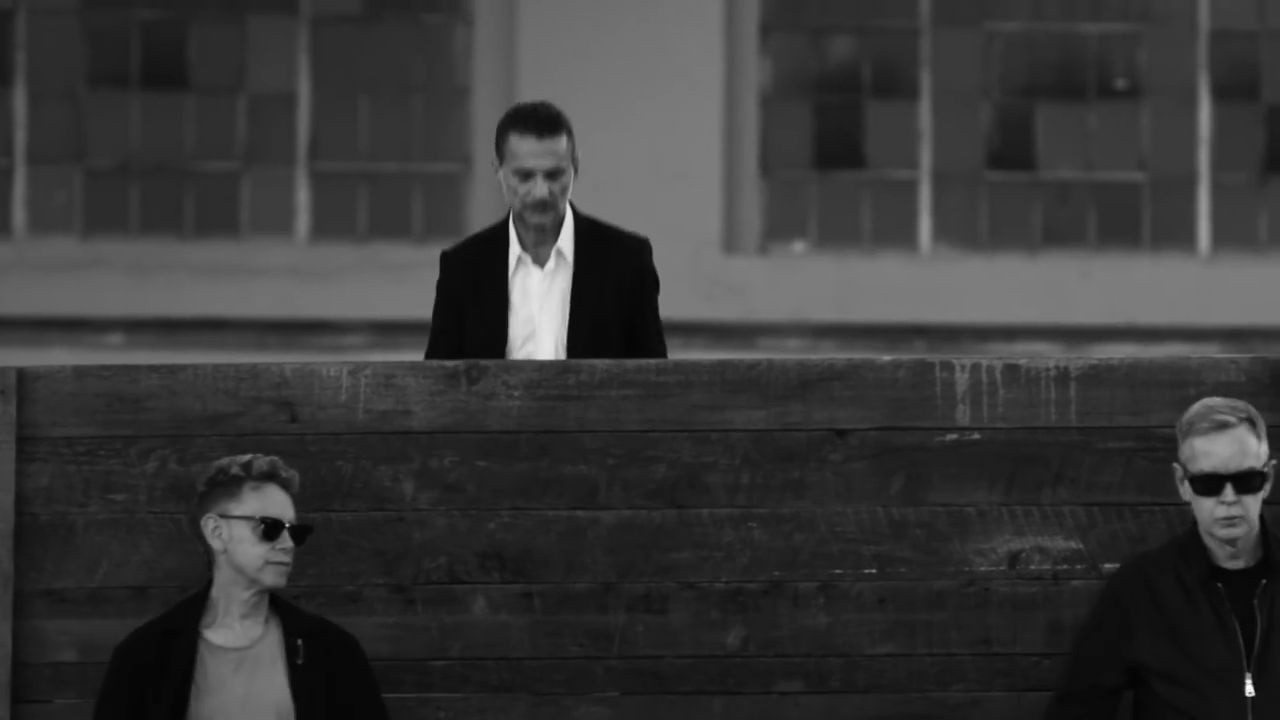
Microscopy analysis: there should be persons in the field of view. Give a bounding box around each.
[93,454,391,720]
[1042,398,1280,720]
[426,101,667,359]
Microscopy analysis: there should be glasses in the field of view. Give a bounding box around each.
[217,512,315,547]
[1176,459,1273,497]
